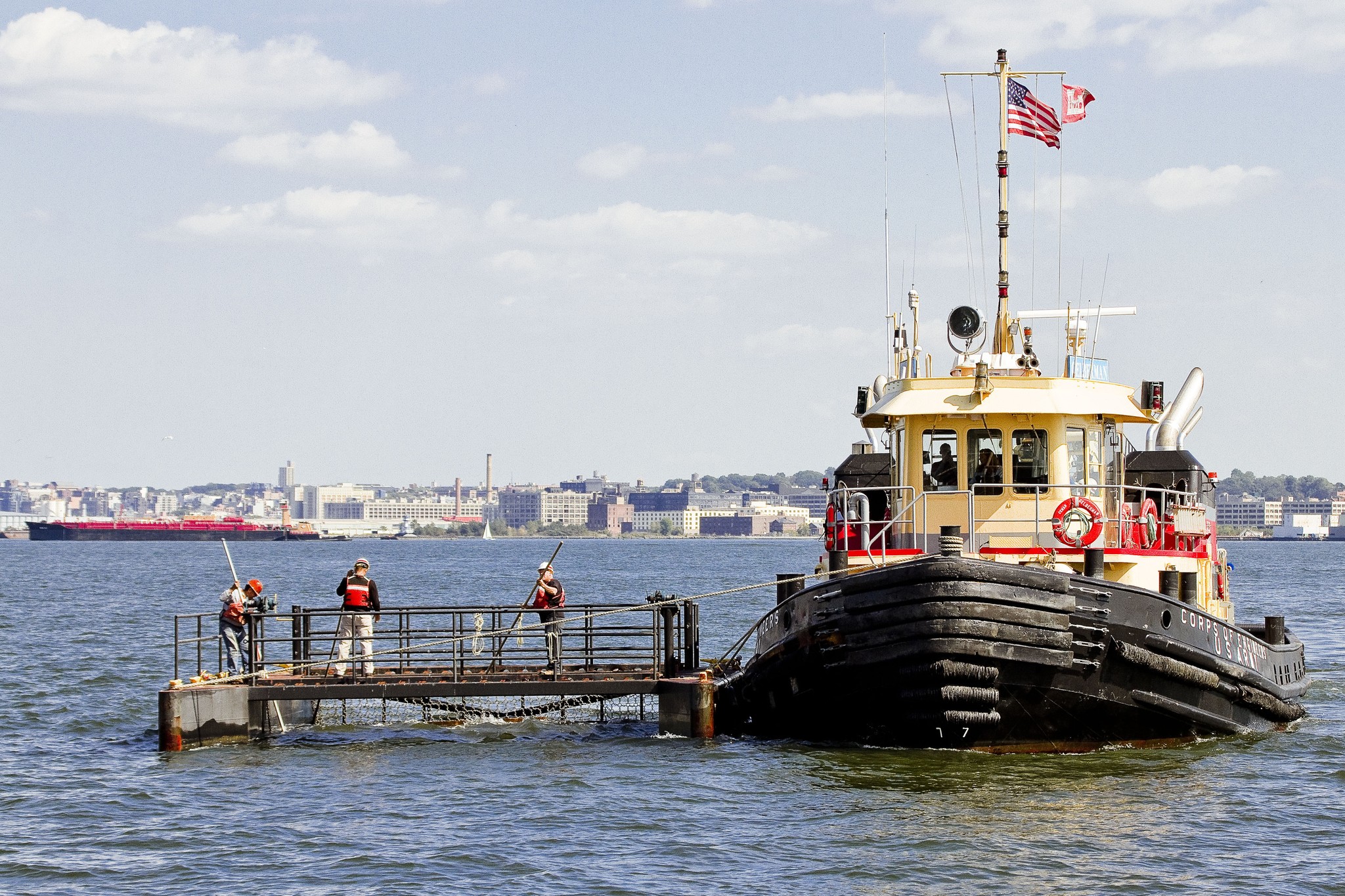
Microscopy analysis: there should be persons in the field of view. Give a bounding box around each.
[931,443,958,485]
[521,562,565,674]
[220,579,262,684]
[968,448,1003,495]
[334,558,381,675]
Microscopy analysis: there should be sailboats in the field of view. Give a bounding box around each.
[482,518,495,542]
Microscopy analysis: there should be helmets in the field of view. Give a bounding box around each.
[355,558,369,566]
[248,579,262,596]
[536,562,553,571]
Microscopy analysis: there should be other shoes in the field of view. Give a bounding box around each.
[365,673,374,678]
[333,674,344,678]
[545,670,558,675]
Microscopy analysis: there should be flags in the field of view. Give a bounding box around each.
[1007,77,1062,150]
[1062,83,1095,124]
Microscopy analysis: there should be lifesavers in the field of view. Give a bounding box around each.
[1138,496,1158,549]
[825,501,835,553]
[1119,505,1134,548]
[1051,498,1103,547]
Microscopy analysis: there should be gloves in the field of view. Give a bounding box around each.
[347,570,354,576]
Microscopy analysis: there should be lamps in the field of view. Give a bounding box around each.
[945,302,988,366]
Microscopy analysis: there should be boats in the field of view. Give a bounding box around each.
[24,491,353,542]
[697,45,1311,759]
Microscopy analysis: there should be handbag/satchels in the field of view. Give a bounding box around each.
[223,603,243,623]
